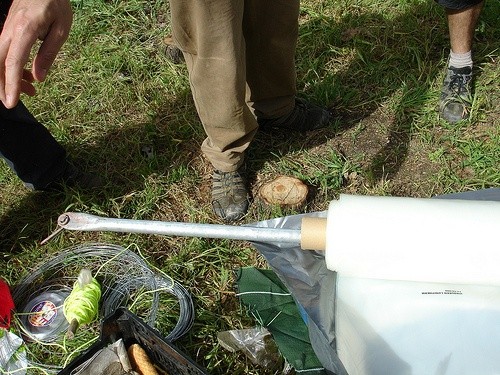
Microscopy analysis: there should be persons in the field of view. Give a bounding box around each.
[435,0,484,122]
[0,0,344,222]
[0,0,79,191]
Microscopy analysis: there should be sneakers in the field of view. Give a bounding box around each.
[439,53,475,123]
[256,97,330,131]
[211,159,249,222]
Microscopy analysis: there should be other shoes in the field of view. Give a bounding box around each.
[40,159,79,207]
